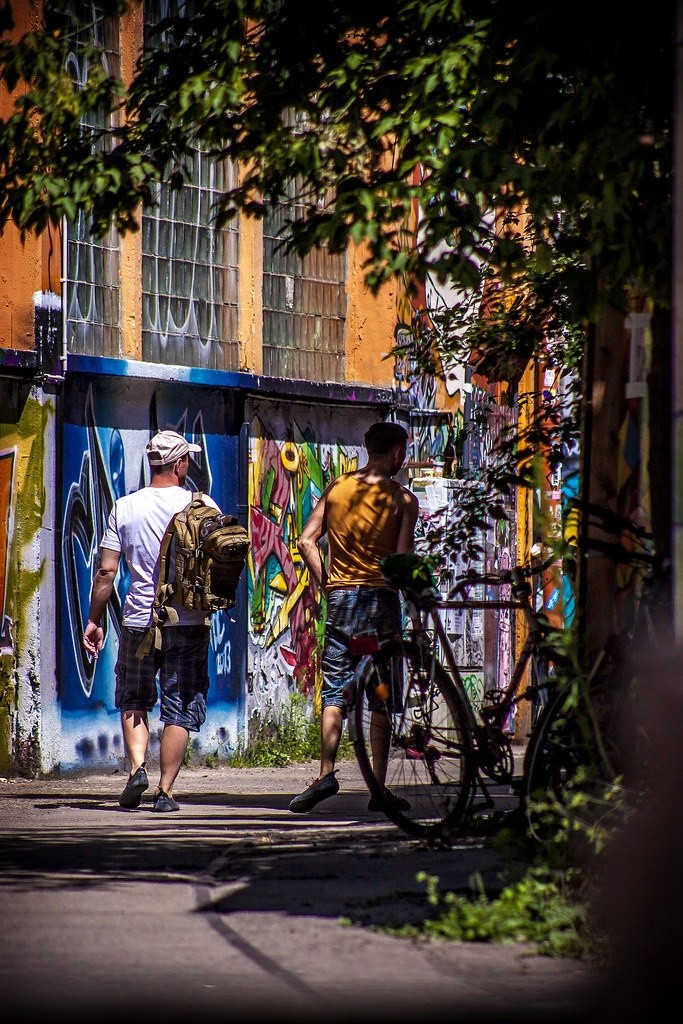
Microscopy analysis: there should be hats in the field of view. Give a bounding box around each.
[146,428,203,465]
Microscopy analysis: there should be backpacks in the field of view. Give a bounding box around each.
[135,491,251,625]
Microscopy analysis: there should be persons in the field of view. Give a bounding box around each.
[285,419,421,812]
[79,428,225,812]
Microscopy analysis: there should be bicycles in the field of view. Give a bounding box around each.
[518,496,683,860]
[343,529,572,843]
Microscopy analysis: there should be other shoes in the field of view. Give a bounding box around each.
[367,795,411,812]
[289,769,340,814]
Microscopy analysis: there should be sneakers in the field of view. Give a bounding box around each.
[119,761,149,809]
[154,785,180,811]
